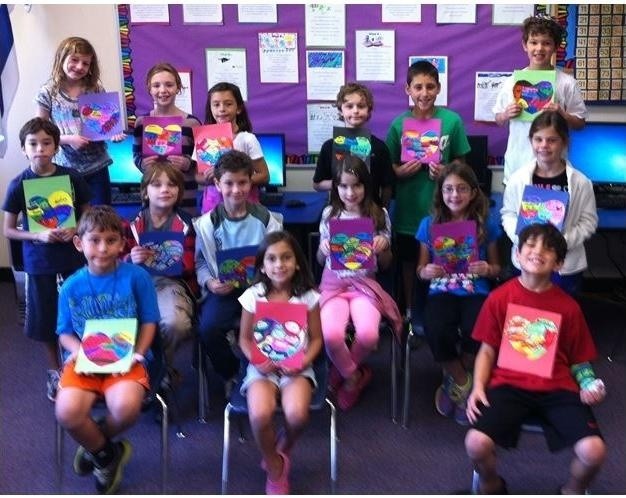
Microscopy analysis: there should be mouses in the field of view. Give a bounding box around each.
[287,199,305,208]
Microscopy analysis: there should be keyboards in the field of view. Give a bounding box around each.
[113,194,142,203]
[257,193,283,206]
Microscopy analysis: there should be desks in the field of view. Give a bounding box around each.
[106,191,330,226]
[491,192,625,228]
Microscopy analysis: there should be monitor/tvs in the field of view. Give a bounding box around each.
[99,134,144,194]
[252,131,286,191]
[567,121,623,185]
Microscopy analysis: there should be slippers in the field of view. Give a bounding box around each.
[259,426,298,470]
[265,451,291,494]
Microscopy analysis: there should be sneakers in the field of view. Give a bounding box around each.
[72,413,109,476]
[46,368,62,401]
[435,367,457,417]
[92,438,131,493]
[447,372,472,425]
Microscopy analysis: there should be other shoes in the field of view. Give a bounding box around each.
[145,370,166,400]
[154,385,174,426]
[337,366,371,411]
[223,372,239,399]
[328,363,343,390]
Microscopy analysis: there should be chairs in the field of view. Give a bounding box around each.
[402,239,424,426]
[472,411,549,493]
[222,352,337,493]
[56,273,170,491]
[307,233,399,424]
[198,343,211,426]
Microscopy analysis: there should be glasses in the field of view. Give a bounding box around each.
[442,183,470,194]
